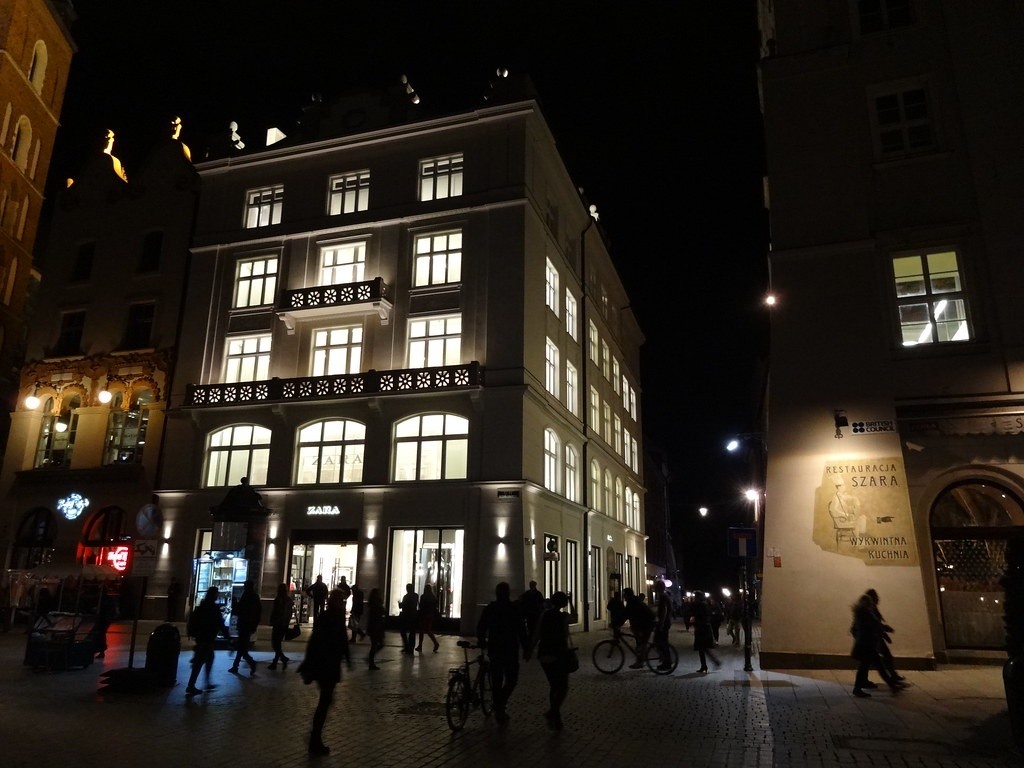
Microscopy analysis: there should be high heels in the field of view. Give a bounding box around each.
[697,666,708,673]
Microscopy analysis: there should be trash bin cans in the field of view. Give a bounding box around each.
[145,622,181,687]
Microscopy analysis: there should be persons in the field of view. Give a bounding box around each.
[295,589,349,754]
[348,584,366,643]
[363,588,387,670]
[681,588,743,673]
[267,583,294,670]
[607,591,626,639]
[849,589,906,697]
[164,577,182,622]
[415,584,440,652]
[306,575,328,623]
[337,577,351,599]
[623,587,655,668]
[517,580,544,642]
[476,582,530,719]
[185,586,230,695]
[83,584,115,658]
[525,591,579,730]
[397,584,418,656]
[118,578,131,619]
[228,580,262,675]
[653,581,673,668]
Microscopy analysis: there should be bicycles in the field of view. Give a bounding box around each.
[446,635,492,731]
[592,625,679,675]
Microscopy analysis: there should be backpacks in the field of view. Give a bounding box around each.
[186,602,213,641]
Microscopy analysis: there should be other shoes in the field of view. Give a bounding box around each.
[657,662,670,672]
[249,659,258,676]
[415,646,422,652]
[186,685,201,694]
[204,683,218,691]
[860,680,875,688]
[433,644,439,653]
[369,663,380,670]
[267,663,277,670]
[852,688,871,700]
[629,660,643,669]
[547,718,560,732]
[94,652,105,661]
[892,683,907,691]
[228,666,240,674]
[400,648,407,652]
[308,743,329,758]
[281,657,290,670]
[896,674,905,680]
[496,713,509,725]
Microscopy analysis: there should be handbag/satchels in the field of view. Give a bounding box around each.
[299,660,313,684]
[284,624,301,642]
[557,647,580,675]
